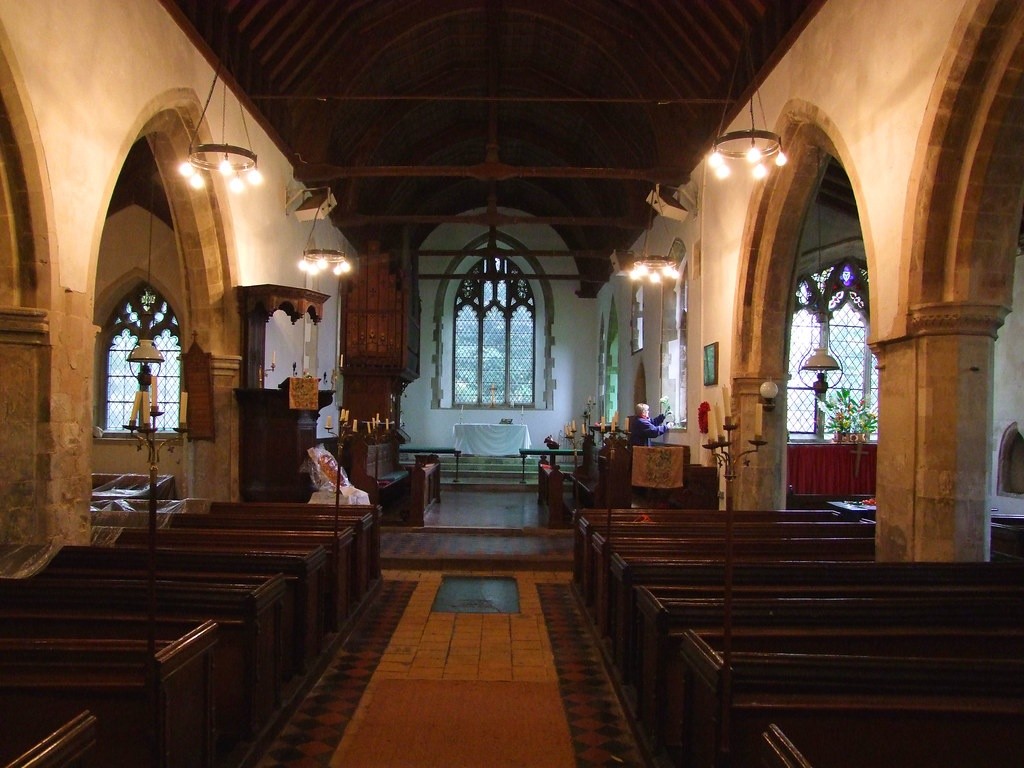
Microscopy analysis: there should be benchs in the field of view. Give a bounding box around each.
[0,474,383,768]
[571,507,1024,768]
[519,448,584,486]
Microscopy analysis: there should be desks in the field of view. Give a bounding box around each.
[758,719,1024,768]
[453,423,532,456]
[828,500,876,521]
[0,695,98,768]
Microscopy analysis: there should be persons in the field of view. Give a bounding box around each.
[626,403,673,508]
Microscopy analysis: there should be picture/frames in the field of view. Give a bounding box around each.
[702,342,720,386]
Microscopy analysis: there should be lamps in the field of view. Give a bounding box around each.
[627,187,682,286]
[297,220,351,276]
[126,154,165,386]
[703,15,787,181]
[760,375,779,412]
[801,192,840,393]
[178,11,263,193]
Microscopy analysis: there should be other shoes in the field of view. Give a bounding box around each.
[631,502,645,509]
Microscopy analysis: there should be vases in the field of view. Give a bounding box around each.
[833,432,866,444]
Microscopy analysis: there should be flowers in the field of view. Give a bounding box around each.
[823,387,878,432]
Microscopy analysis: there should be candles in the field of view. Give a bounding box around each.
[521,406,523,414]
[341,354,343,367]
[327,409,388,434]
[707,387,763,439]
[565,412,629,437]
[461,405,463,413]
[272,352,275,363]
[131,376,188,423]
